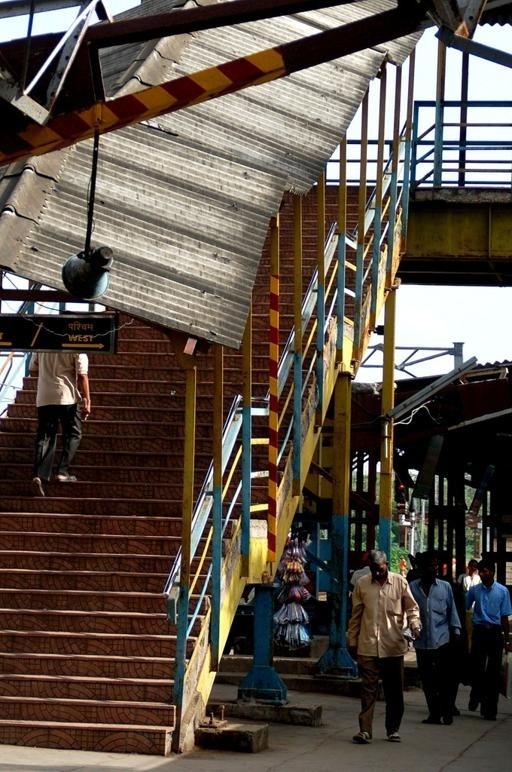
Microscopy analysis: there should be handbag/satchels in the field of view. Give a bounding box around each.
[499,652,512,699]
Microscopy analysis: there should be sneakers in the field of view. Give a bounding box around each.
[30,476,46,496]
[353,732,373,743]
[55,474,77,481]
[388,732,401,742]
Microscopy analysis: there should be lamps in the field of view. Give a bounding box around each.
[60,130,115,303]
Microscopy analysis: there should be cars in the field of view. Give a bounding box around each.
[421,550,470,580]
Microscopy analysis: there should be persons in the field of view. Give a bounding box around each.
[347,551,483,655]
[407,550,465,727]
[345,546,424,744]
[460,557,512,722]
[25,349,93,499]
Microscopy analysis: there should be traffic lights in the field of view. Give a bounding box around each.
[395,473,409,502]
